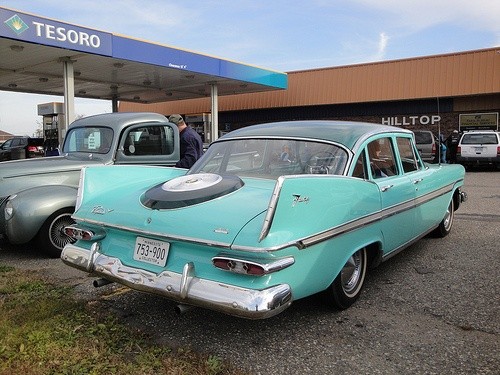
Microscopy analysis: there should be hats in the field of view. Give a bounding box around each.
[168,114,184,125]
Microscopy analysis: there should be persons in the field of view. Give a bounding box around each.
[280,145,291,161]
[432,130,461,163]
[168,114,204,169]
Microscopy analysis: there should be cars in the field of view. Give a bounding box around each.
[0,135,46,161]
[399,129,437,163]
[456,131,500,171]
[60,119,468,322]
[0,111,181,254]
